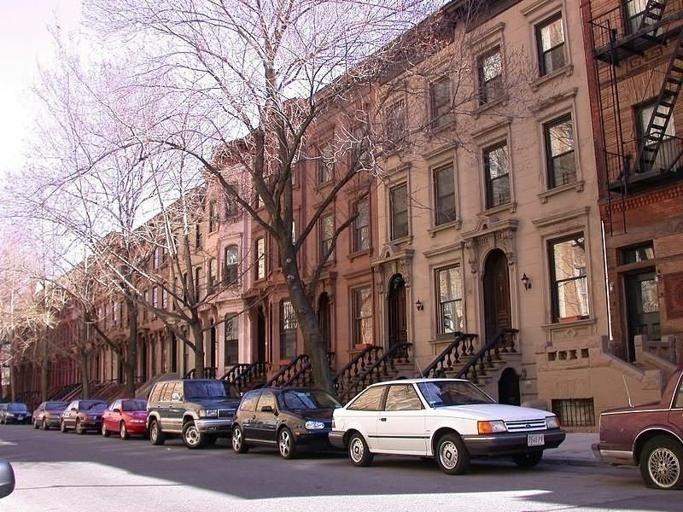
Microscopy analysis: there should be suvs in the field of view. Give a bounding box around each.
[143,379,240,449]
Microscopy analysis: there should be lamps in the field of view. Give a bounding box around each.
[415,299,423,312]
[521,274,532,290]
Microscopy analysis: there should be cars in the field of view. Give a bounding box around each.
[327,378,566,476]
[1,398,149,440]
[590,367,683,492]
[231,387,344,459]
